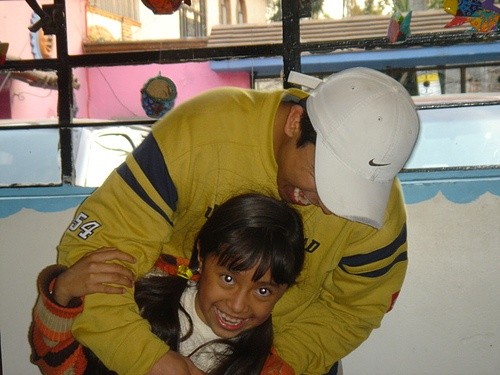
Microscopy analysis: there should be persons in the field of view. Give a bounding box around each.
[54,67,422,375]
[26,192,308,375]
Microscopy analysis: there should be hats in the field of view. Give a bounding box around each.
[307,66,420,231]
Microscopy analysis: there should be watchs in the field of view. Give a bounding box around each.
[46,274,80,309]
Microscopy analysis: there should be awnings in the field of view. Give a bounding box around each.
[209,40,499,76]
[206,9,478,38]
